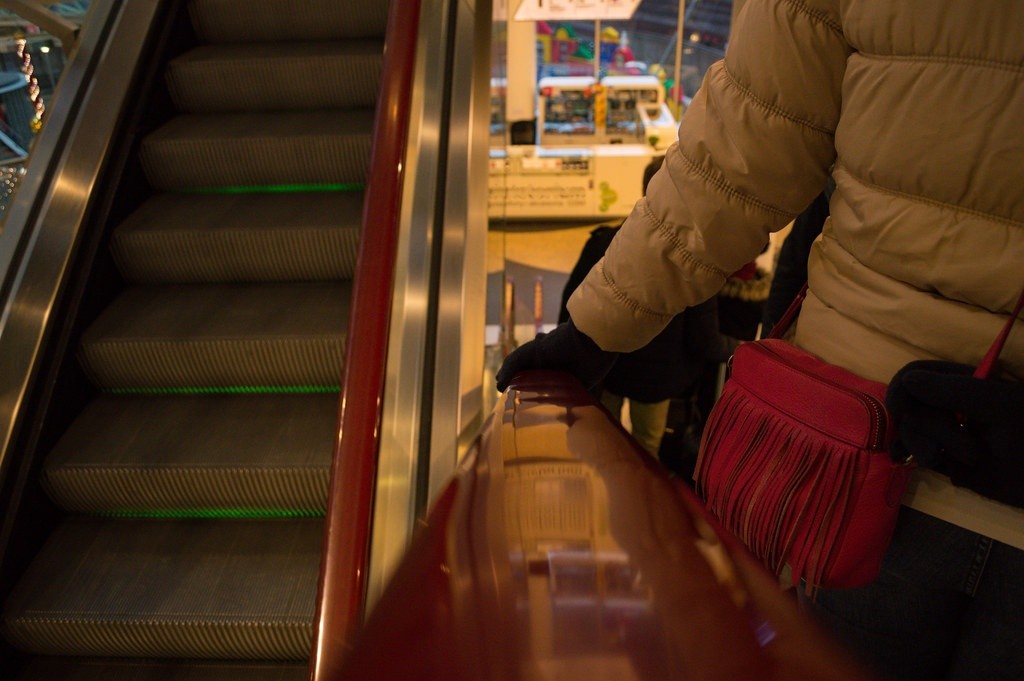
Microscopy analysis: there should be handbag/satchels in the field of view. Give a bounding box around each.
[693,340,921,600]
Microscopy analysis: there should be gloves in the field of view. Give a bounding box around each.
[886,361,1024,513]
[496,318,619,394]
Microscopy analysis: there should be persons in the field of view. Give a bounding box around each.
[495,0,1024,681]
[553,152,840,486]
